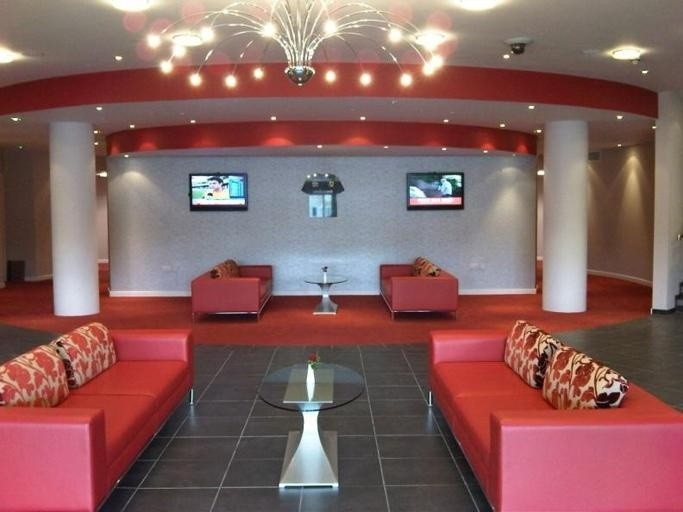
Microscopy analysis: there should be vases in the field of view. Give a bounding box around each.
[323,273,328,284]
[305,365,315,402]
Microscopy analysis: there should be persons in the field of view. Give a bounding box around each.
[207,177,229,200]
[439,178,453,197]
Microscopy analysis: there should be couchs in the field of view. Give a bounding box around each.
[190,264,273,329]
[378,264,460,322]
[0,329,194,512]
[425,328,683,512]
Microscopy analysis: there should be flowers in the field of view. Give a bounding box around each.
[305,350,320,371]
[320,266,328,272]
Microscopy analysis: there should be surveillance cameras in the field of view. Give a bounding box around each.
[505,38,534,55]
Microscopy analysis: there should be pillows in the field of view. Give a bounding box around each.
[0,343,71,408]
[502,319,564,388]
[48,319,117,389]
[542,344,630,411]
[211,259,242,280]
[412,256,441,278]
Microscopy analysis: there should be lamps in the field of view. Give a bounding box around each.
[146,0,457,89]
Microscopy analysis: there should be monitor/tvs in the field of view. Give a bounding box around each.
[189,173,248,212]
[406,173,464,210]
